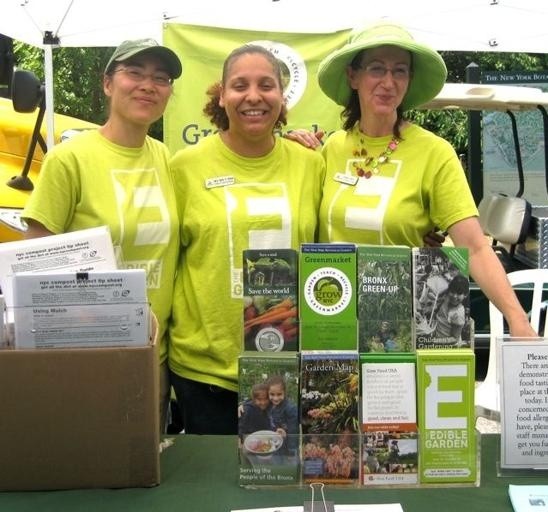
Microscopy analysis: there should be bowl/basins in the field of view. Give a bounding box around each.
[244,431,285,457]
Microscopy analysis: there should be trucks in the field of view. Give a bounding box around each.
[1,65,106,247]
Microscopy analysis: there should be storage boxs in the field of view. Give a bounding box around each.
[2,304,160,489]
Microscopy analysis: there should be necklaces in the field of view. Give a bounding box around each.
[351,129,399,178]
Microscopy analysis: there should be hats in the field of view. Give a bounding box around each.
[102,37,183,80]
[317,20,449,111]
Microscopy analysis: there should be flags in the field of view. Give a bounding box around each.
[163,22,352,155]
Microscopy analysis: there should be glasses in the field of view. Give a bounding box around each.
[110,67,173,85]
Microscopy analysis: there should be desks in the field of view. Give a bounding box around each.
[0,429,548,511]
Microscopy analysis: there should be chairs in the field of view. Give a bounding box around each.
[476,269,548,423]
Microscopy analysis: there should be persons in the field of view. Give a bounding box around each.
[19,37,182,435]
[416,265,469,348]
[238,383,271,467]
[283,22,540,342]
[238,375,298,468]
[166,44,327,435]
